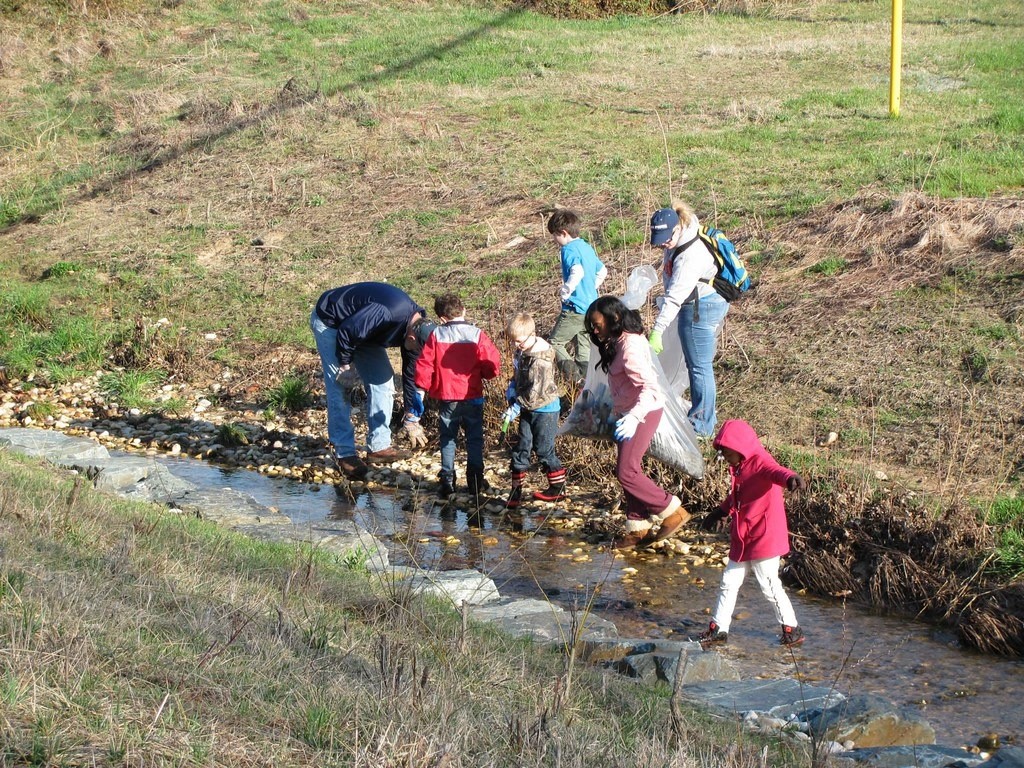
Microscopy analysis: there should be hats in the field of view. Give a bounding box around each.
[650,208,678,245]
[415,320,437,348]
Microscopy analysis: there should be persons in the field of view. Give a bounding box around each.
[501,313,567,509]
[311,282,437,478]
[408,295,501,496]
[584,295,691,550]
[548,210,607,390]
[687,419,806,647]
[648,201,730,438]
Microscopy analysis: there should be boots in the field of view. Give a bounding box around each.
[655,494,693,542]
[507,473,526,506]
[616,518,655,550]
[534,468,566,501]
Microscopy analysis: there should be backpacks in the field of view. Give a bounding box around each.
[671,227,750,302]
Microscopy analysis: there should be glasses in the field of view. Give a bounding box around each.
[661,230,675,247]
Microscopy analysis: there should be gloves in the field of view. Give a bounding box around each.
[649,330,663,355]
[409,386,425,417]
[501,401,522,422]
[701,506,726,531]
[614,413,639,442]
[335,362,355,387]
[787,475,806,493]
[506,381,516,400]
[398,417,429,448]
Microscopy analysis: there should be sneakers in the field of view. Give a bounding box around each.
[771,624,805,646]
[687,621,727,645]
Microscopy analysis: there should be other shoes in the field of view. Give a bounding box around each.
[438,476,457,493]
[339,456,368,477]
[468,476,489,495]
[366,448,412,463]
